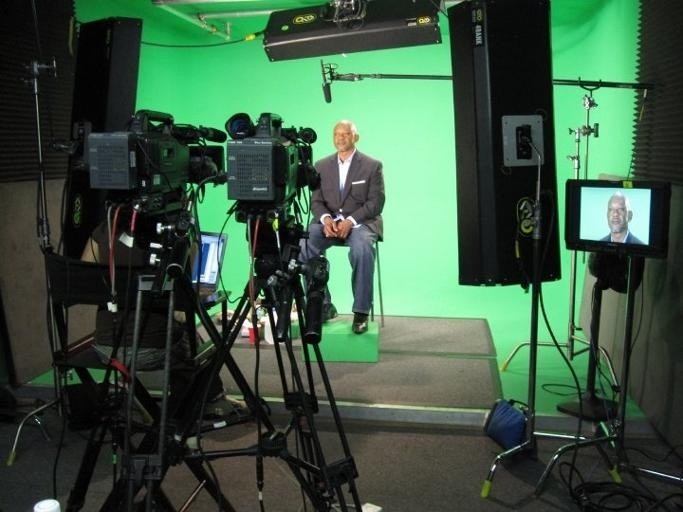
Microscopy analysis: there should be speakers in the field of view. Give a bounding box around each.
[63,18,143,259]
[449,0,562,286]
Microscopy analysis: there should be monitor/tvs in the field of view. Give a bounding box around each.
[566,179,671,260]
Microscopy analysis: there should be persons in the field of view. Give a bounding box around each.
[597,193,646,246]
[298,121,384,334]
[78,205,247,418]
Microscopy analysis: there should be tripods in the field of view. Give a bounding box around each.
[481,134,623,499]
[534,259,683,495]
[8,66,150,466]
[172,272,360,512]
[63,274,328,512]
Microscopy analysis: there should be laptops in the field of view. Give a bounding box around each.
[192,232,226,298]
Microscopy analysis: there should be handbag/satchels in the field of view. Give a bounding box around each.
[484,399,529,448]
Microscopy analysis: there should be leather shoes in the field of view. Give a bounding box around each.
[321,303,338,322]
[353,314,368,333]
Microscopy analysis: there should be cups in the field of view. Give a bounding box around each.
[247,326,258,345]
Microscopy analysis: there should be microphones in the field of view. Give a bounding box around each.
[324,84,331,104]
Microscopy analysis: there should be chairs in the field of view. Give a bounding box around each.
[43,247,196,369]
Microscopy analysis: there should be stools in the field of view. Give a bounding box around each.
[325,237,386,325]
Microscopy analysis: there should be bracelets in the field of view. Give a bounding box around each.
[345,219,352,224]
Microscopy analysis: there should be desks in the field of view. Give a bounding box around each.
[175,289,230,368]
